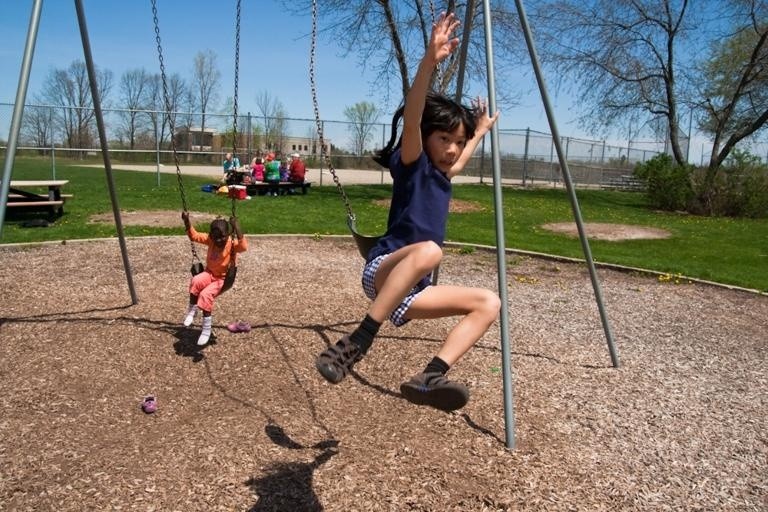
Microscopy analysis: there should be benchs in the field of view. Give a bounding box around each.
[7,200,65,227]
[254,181,312,196]
[7,193,74,217]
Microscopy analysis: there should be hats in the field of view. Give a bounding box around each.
[291,153,300,158]
[266,154,275,160]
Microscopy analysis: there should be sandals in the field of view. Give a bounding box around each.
[224,322,252,333]
[140,398,159,412]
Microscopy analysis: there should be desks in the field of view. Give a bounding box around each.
[231,168,266,192]
[9,179,69,219]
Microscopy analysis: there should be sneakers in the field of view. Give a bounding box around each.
[400,371,470,412]
[317,336,364,385]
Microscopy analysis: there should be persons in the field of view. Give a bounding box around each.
[287,151,307,194]
[180,211,249,347]
[249,148,264,166]
[222,151,240,185]
[278,160,289,196]
[249,156,265,180]
[263,153,281,197]
[315,6,501,412]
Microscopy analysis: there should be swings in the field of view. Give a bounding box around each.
[306,0,445,263]
[149,0,241,293]
